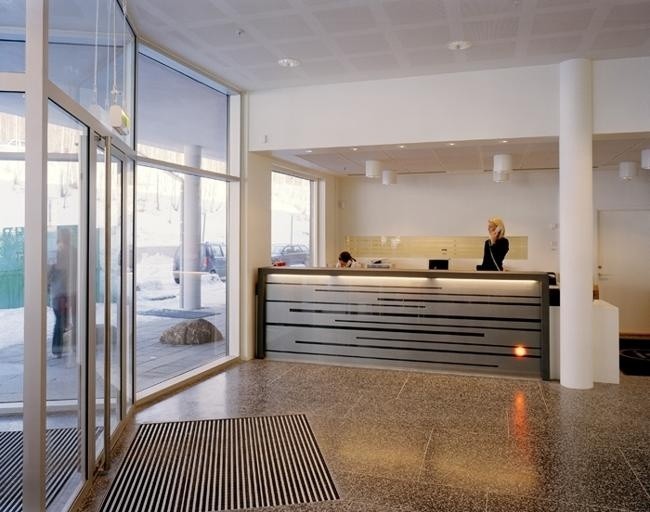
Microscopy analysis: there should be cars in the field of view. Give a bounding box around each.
[271,242,311,267]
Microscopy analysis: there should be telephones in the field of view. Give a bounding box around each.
[495,227,501,233]
[346,260,351,267]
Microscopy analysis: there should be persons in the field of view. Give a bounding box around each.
[336,251,361,269]
[47,243,70,359]
[482,217,509,270]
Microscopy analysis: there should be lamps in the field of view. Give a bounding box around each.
[365,148,650,185]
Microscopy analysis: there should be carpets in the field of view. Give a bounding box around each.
[619,348,650,376]
[0,426,104,507]
[99,413,340,512]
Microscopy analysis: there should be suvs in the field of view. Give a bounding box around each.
[171,241,226,287]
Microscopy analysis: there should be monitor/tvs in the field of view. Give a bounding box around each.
[429,259,448,269]
